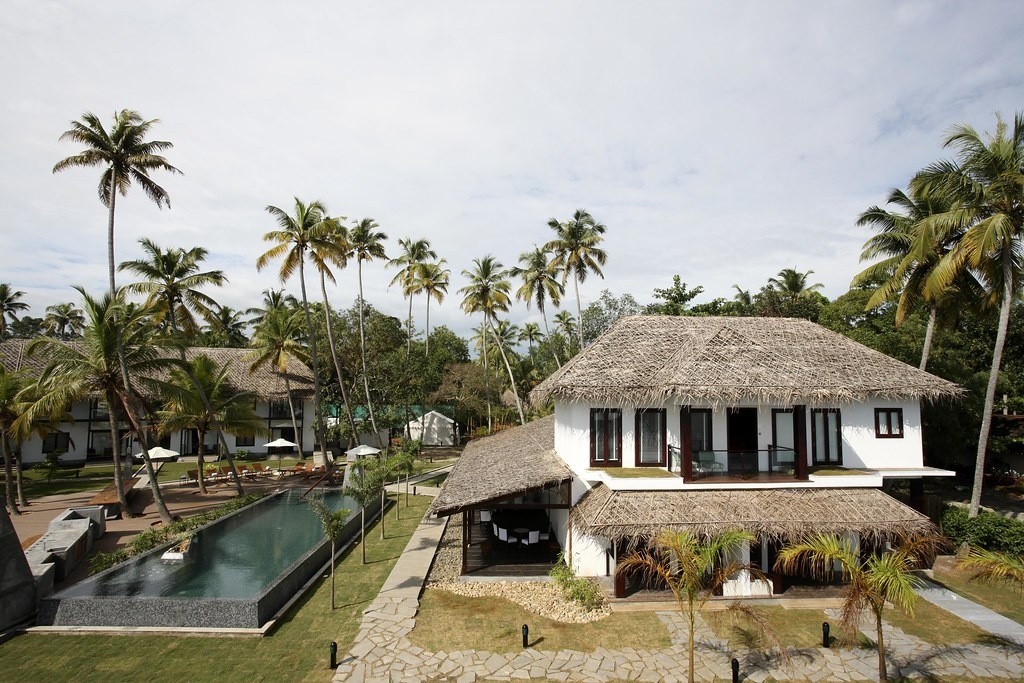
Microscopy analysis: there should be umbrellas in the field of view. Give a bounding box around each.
[134,447,180,481]
[262,437,299,475]
[344,445,382,460]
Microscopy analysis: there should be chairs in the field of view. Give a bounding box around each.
[203,444,220,456]
[180,461,341,488]
[668,448,724,477]
[479,491,552,549]
[88,447,113,459]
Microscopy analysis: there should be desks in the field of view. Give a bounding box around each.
[513,527,529,549]
[277,468,289,482]
